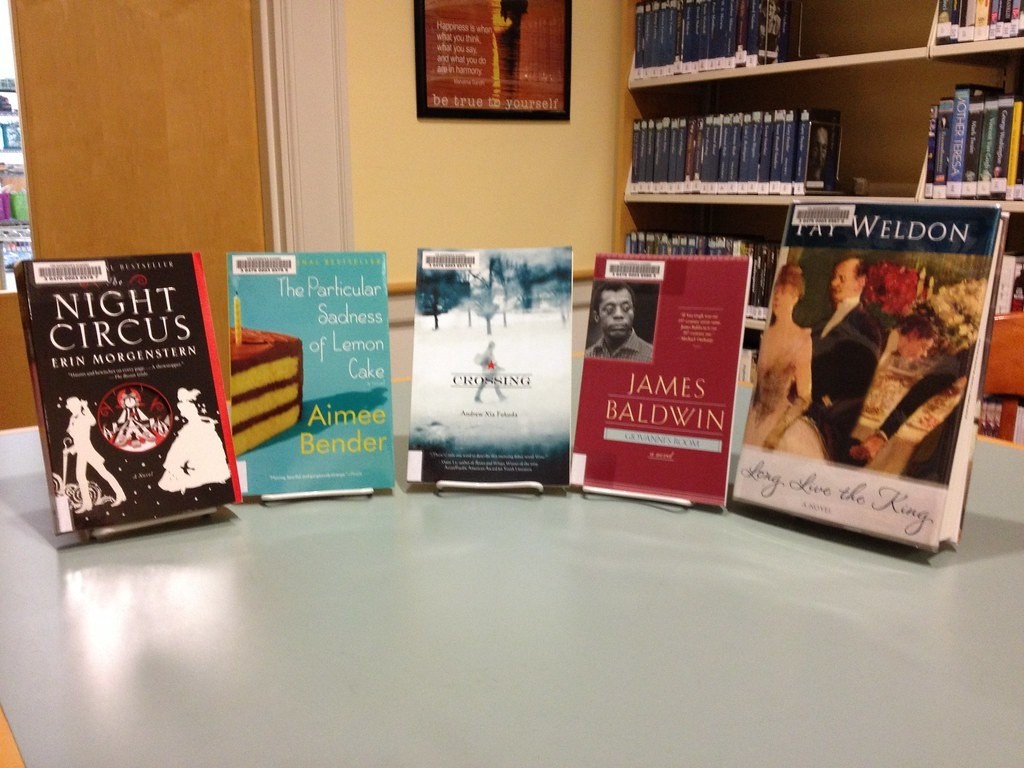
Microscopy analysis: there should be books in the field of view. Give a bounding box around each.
[226,250,396,496]
[732,197,1009,553]
[571,251,753,508]
[928,84,1024,202]
[971,250,1024,445]
[936,0,1024,45]
[629,107,845,197]
[631,0,792,80]
[0,78,35,272]
[625,228,781,385]
[406,248,574,489]
[14,252,242,536]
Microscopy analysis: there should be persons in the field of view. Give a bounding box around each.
[808,124,830,188]
[583,280,655,362]
[742,257,978,487]
[754,1,781,64]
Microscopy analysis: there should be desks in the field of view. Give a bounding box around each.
[0,355,1024,768]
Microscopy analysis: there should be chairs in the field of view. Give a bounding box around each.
[982,311,1024,441]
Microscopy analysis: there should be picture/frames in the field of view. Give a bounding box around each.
[414,0,573,121]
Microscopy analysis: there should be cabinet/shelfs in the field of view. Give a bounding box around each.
[0,78,34,290]
[622,0,1024,332]
